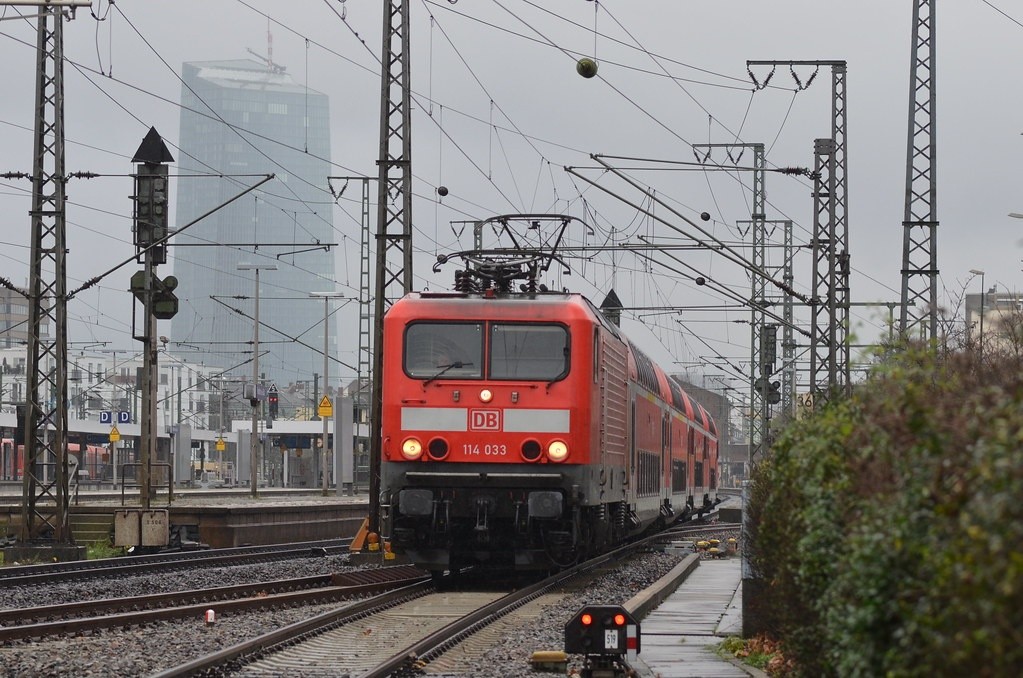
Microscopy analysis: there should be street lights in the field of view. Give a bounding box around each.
[237,262,278,496]
[969,269,984,360]
[310,291,344,496]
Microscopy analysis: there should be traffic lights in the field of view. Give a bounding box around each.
[269,392,278,419]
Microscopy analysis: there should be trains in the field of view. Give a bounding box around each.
[380,257,721,576]
